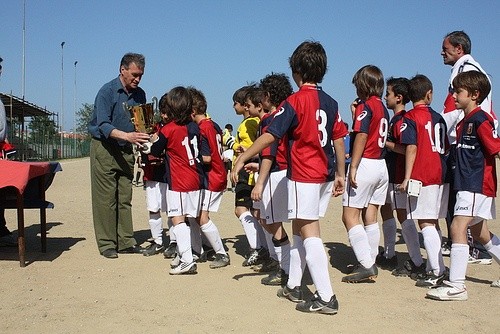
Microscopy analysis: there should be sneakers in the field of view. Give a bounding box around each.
[261,269,289,285]
[441,242,451,255]
[253,258,278,271]
[143,241,165,255]
[296,291,338,314]
[391,259,426,276]
[416,272,449,286]
[277,286,303,302]
[171,256,180,267]
[426,280,467,300]
[242,246,267,266]
[163,243,177,257]
[169,261,197,275]
[342,262,378,282]
[376,251,397,269]
[492,280,500,286]
[411,271,426,280]
[468,247,492,264]
[198,249,215,261]
[208,253,230,268]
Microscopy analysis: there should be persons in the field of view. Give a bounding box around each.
[149,86,204,276]
[88,52,147,259]
[89,31,497,316]
[340,64,390,283]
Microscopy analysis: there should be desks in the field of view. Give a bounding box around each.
[0,159,63,268]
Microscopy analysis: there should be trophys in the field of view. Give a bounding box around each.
[393,179,422,197]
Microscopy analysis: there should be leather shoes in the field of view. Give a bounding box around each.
[120,244,146,253]
[101,249,118,258]
